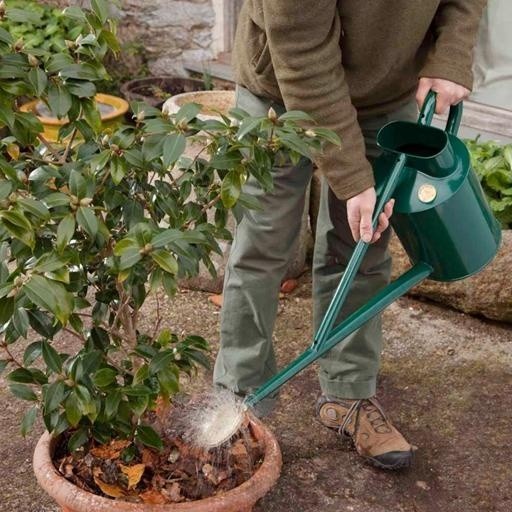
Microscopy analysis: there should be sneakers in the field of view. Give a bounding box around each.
[315,395,413,470]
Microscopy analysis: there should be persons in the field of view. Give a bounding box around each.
[213,3,491,468]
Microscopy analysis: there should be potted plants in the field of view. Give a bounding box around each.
[0,1,344,511]
